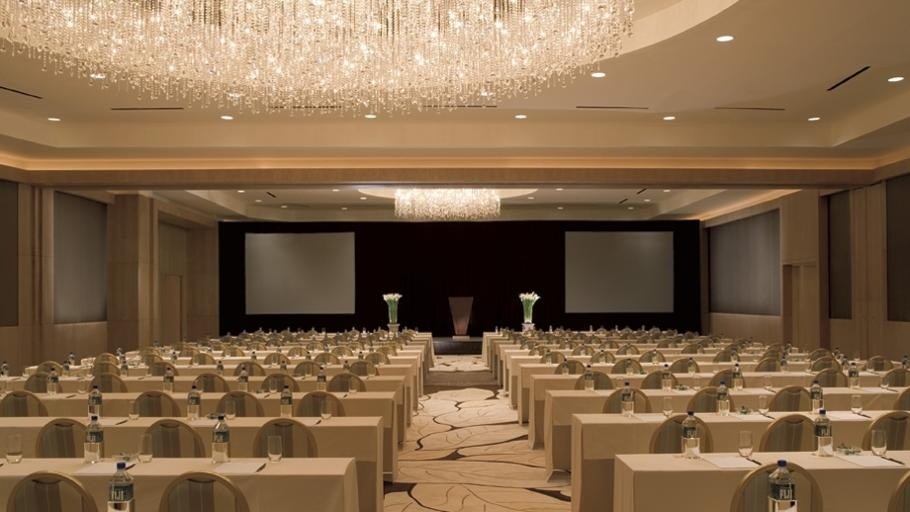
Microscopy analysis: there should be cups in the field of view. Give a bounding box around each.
[84,440,101,462]
[320,399,333,419]
[693,375,703,392]
[136,434,153,462]
[817,436,833,457]
[621,401,634,419]
[737,430,754,457]
[879,376,890,390]
[614,377,623,390]
[0,332,375,395]
[224,400,237,420]
[661,396,674,416]
[850,395,864,414]
[757,395,771,415]
[267,436,283,462]
[870,430,888,456]
[684,437,700,462]
[87,405,99,421]
[761,376,775,391]
[211,442,228,462]
[128,401,140,420]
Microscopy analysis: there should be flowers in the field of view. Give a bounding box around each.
[383,293,403,324]
[518,291,542,324]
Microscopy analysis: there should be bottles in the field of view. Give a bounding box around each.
[731,363,744,391]
[86,385,103,405]
[524,333,909,375]
[87,415,102,457]
[620,381,634,418]
[660,364,672,391]
[185,386,200,423]
[681,411,698,453]
[584,364,595,392]
[211,415,230,460]
[279,385,292,418]
[810,380,822,414]
[766,461,796,511]
[715,381,730,416]
[109,463,134,512]
[814,408,831,456]
[848,361,860,388]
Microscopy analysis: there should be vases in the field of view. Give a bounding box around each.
[387,302,399,324]
[524,304,533,324]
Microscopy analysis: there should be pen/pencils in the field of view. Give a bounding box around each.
[115,420,128,425]
[882,456,904,465]
[746,457,762,466]
[861,414,871,418]
[766,416,775,419]
[316,420,321,424]
[256,463,266,472]
[124,464,136,470]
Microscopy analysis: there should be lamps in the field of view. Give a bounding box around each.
[1,0,634,116]
[394,187,500,220]
